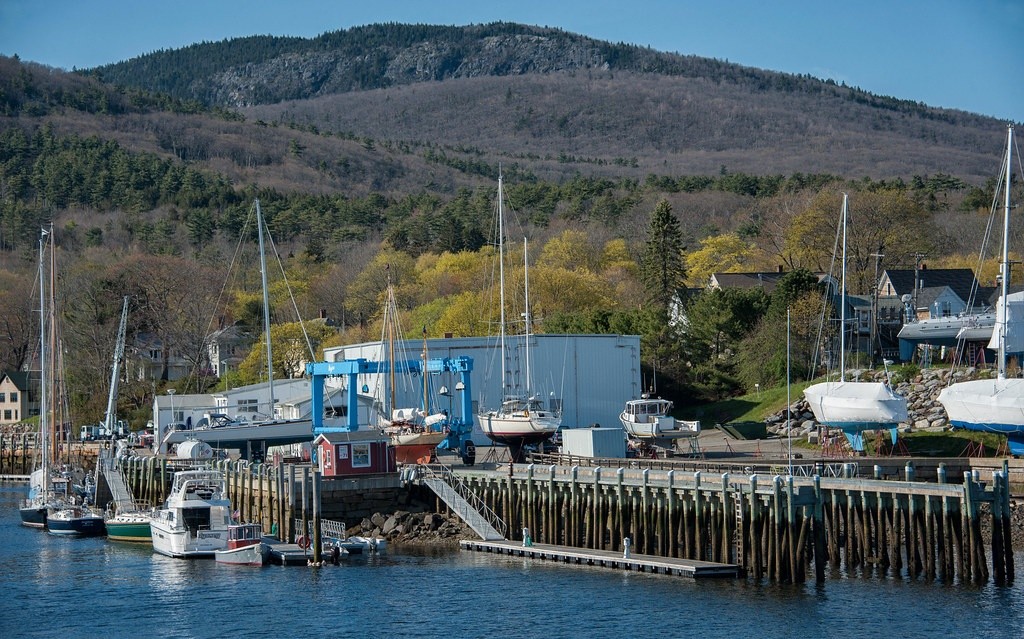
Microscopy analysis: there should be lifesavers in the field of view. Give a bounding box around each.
[298,536,310,549]
[524,410,529,417]
[406,428,412,434]
[211,492,221,501]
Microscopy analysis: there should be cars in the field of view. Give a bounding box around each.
[80,414,268,452]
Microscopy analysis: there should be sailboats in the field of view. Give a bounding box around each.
[477,161,564,463]
[937,120,1023,456]
[360,264,447,471]
[893,118,1024,364]
[161,194,348,448]
[15,219,106,540]
[800,190,908,453]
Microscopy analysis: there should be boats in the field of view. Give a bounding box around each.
[617,359,700,461]
[154,469,259,569]
[104,499,156,544]
[211,541,272,568]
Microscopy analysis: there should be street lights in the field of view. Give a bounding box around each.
[168,389,176,428]
